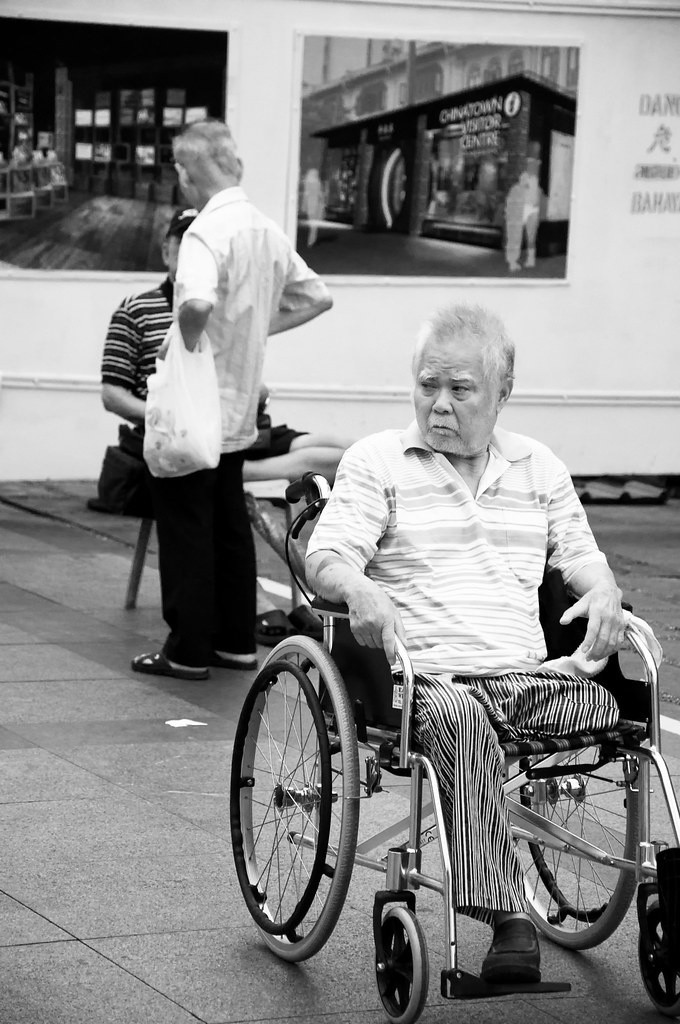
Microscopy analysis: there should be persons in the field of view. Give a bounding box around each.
[101,210,355,481]
[304,307,626,984]
[131,122,333,680]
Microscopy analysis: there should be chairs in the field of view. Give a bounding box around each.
[125,477,303,613]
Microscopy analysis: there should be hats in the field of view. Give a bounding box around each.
[165,207,199,237]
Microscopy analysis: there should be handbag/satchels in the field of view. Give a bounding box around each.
[143,322,221,478]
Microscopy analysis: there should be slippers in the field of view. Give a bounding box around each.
[131,653,209,679]
[206,648,258,671]
[254,609,300,646]
[287,605,324,639]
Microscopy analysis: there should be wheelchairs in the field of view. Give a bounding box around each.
[228,469,680,1024]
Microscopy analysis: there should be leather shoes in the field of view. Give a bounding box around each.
[480,918,541,985]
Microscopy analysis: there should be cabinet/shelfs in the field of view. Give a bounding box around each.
[72,84,190,182]
